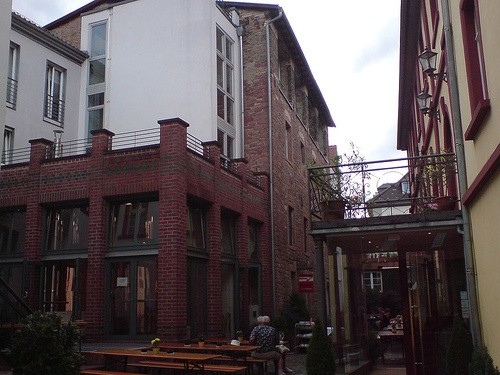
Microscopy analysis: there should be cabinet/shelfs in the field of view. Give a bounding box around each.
[295,322,313,353]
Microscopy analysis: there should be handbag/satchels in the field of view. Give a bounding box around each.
[275,344,291,353]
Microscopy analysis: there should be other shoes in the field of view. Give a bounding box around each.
[283,367,293,372]
[277,369,287,375]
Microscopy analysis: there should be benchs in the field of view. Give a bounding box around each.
[1,320,89,347]
[79,355,270,375]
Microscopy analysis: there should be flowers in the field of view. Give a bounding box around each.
[151,337,161,348]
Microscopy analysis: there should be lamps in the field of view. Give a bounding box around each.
[417,45,448,84]
[417,87,441,123]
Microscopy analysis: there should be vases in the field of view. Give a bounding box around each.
[153,347,160,354]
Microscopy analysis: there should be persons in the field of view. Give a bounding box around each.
[251,316,293,375]
[379,309,390,329]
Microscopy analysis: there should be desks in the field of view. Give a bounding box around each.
[89,349,222,375]
[204,337,249,345]
[378,330,405,366]
[149,342,261,375]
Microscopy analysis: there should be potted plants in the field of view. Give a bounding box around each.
[199,336,204,347]
[306,155,353,221]
[421,147,458,211]
